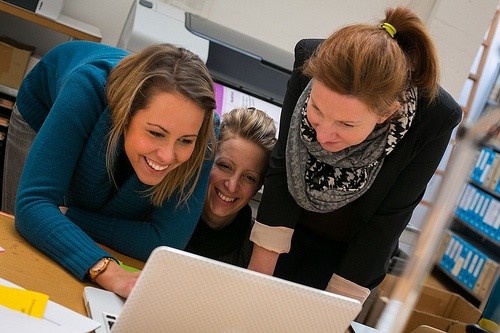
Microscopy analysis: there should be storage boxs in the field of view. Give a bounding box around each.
[365,275,485,333]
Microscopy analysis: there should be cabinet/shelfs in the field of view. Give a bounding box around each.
[433,65,500,326]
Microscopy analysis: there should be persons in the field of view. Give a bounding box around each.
[2,39,221,299]
[183,107,276,269]
[246,7,462,306]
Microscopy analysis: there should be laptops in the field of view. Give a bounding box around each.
[81,245,362,333]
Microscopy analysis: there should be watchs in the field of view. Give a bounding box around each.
[87,258,111,281]
